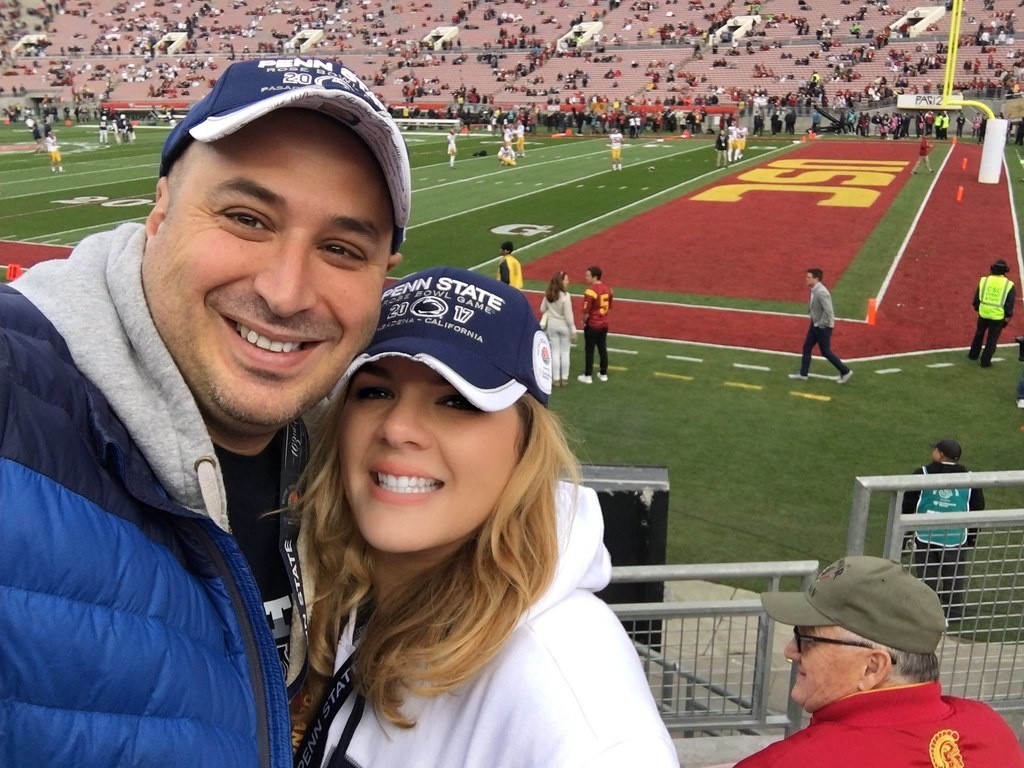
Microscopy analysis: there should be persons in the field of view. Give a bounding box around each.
[902,439,985,621]
[705,558,1024,768]
[498,241,524,291]
[0,0,1024,174]
[965,260,1016,368]
[289,265,684,768]
[540,270,577,386]
[1014,369,1024,408]
[577,267,614,383]
[788,269,854,384]
[0,50,412,768]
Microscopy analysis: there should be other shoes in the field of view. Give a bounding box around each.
[59,167,65,172]
[553,380,560,387]
[597,372,608,381]
[561,379,569,386]
[52,168,57,173]
[578,373,593,384]
[789,374,808,381]
[837,370,853,384]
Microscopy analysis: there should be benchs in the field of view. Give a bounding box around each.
[0,0,1024,103]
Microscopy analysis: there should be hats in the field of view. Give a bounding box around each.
[159,57,410,255]
[328,266,552,412]
[930,440,961,462]
[761,555,944,654]
[501,241,513,252]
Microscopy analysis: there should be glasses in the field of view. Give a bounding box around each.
[794,627,898,665]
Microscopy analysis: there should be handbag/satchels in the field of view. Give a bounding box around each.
[540,311,547,330]
